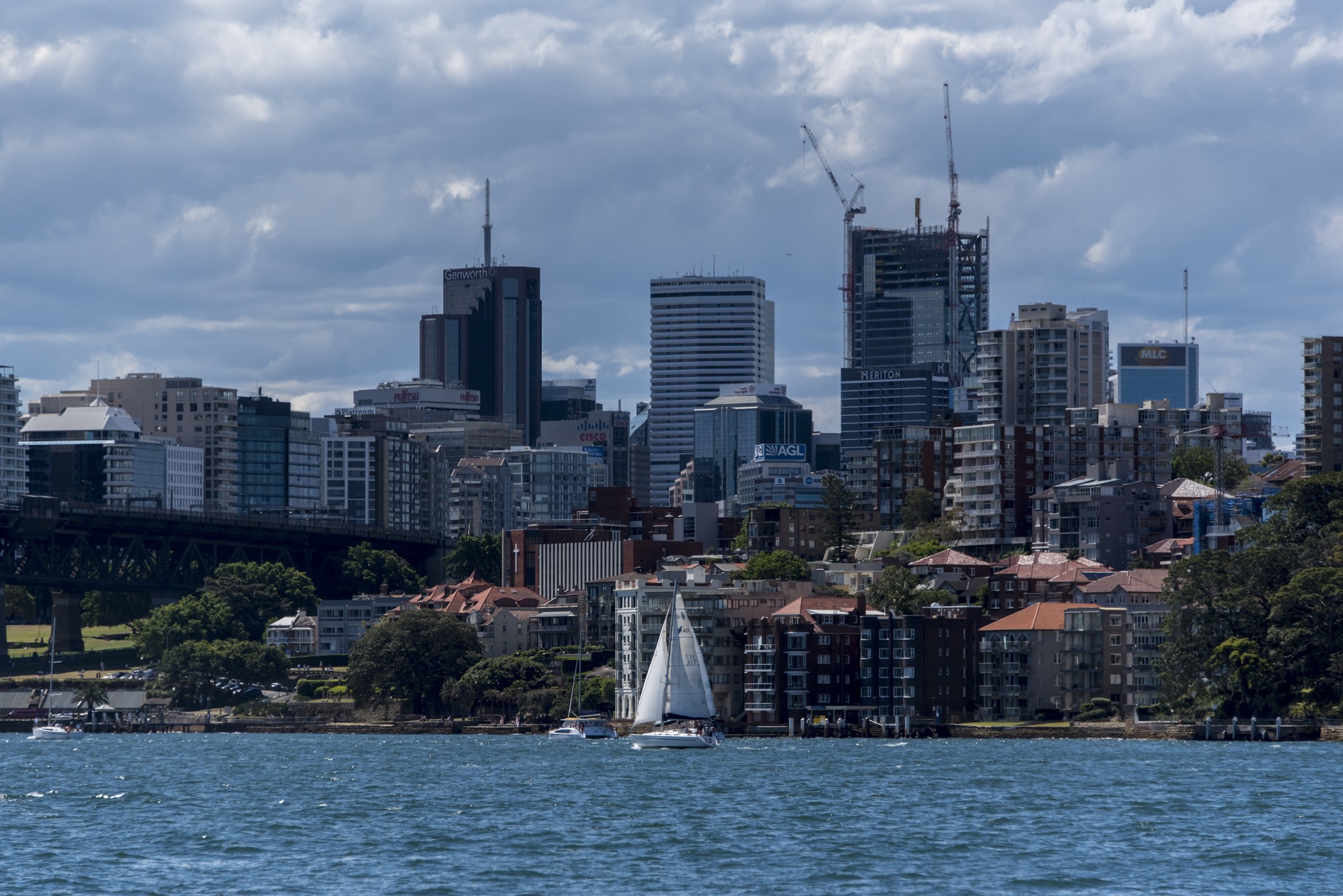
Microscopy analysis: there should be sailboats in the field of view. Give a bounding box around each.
[548,618,620,740]
[627,577,726,750]
[31,617,86,740]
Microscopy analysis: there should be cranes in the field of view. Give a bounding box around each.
[941,82,964,426]
[800,122,868,368]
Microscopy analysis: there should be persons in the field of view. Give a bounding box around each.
[698,721,706,735]
[686,729,693,734]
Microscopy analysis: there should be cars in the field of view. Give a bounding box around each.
[204,675,297,699]
[102,668,158,681]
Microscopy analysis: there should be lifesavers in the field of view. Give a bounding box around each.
[1285,718,1290,723]
[609,724,612,729]
[65,726,71,732]
[710,726,717,734]
